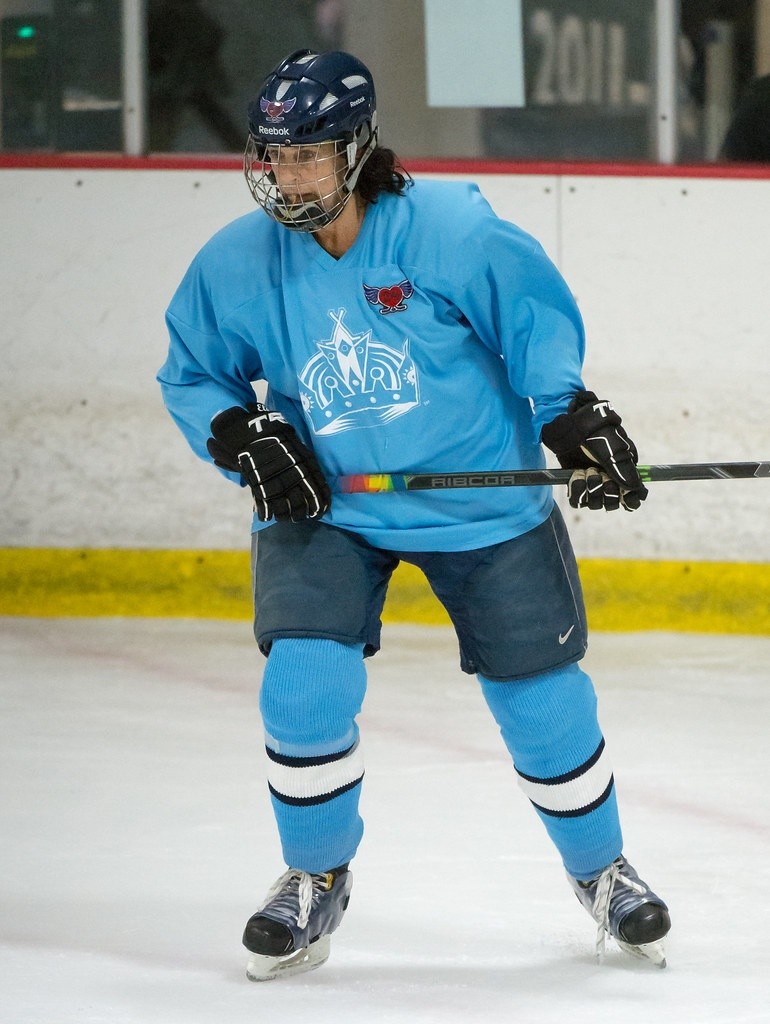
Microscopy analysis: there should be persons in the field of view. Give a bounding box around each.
[155,48,673,983]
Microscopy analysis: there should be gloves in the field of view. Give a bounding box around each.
[206,402,330,524]
[539,390,648,514]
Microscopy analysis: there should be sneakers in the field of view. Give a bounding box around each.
[571,859,669,969]
[244,862,354,982]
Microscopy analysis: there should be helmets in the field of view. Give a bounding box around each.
[243,48,378,234]
